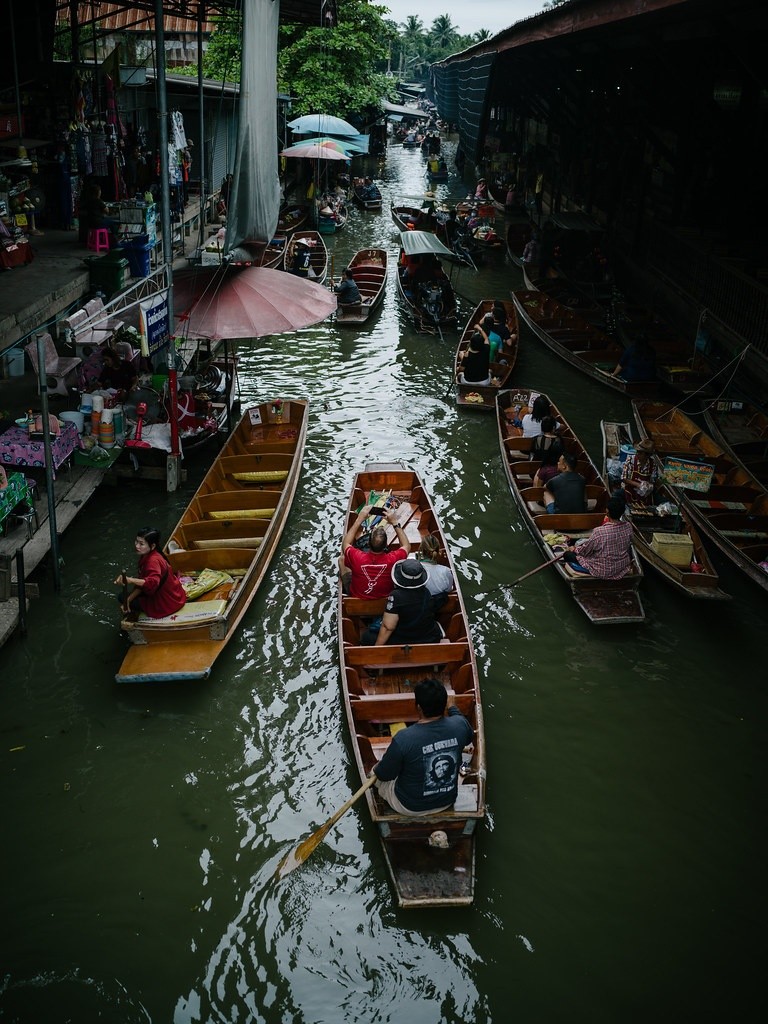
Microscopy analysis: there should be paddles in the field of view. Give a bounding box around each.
[120,569,132,618]
[502,550,568,589]
[445,371,462,396]
[275,777,376,883]
[329,254,337,321]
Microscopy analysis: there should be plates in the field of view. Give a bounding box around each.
[193,390,221,401]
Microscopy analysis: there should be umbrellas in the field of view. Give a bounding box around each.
[280,112,370,195]
[172,264,337,432]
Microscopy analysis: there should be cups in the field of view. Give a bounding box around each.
[100,409,113,425]
[92,397,104,413]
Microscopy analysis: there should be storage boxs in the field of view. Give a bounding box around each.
[652,533,694,566]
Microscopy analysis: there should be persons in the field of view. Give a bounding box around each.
[338,504,412,601]
[113,527,186,622]
[520,232,544,263]
[505,183,526,217]
[360,178,377,199]
[459,300,517,387]
[81,184,109,219]
[330,267,362,304]
[563,495,633,579]
[427,154,447,173]
[397,102,440,142]
[292,237,311,268]
[415,535,454,596]
[534,167,545,216]
[475,177,487,198]
[424,134,440,150]
[520,395,588,514]
[612,335,657,383]
[398,249,442,284]
[369,678,475,817]
[364,558,440,647]
[445,209,463,247]
[221,174,233,210]
[123,148,142,197]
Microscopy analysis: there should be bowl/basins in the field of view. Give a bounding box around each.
[15,418,29,428]
[59,409,123,449]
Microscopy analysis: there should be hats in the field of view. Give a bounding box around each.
[391,559,429,589]
[633,438,656,454]
[509,184,516,192]
[321,206,333,214]
[437,204,450,212]
[424,192,435,202]
[477,178,487,185]
[295,237,308,247]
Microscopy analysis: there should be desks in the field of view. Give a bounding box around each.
[0,420,85,487]
[0,472,33,538]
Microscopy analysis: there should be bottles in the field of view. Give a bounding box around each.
[28,410,36,439]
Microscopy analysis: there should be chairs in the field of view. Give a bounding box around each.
[26,333,83,396]
[83,296,125,331]
[64,309,113,360]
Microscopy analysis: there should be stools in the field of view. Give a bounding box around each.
[4,505,40,538]
[26,478,41,506]
[87,228,109,253]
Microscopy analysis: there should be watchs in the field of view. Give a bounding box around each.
[393,523,401,528]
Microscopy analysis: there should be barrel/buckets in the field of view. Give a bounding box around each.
[79,394,93,413]
[181,376,195,393]
[618,444,637,462]
[152,375,169,396]
[7,348,24,376]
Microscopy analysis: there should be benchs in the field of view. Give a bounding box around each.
[564,562,634,578]
[709,513,768,538]
[503,436,574,459]
[181,517,271,547]
[684,486,758,510]
[221,454,294,482]
[168,546,257,576]
[370,784,479,816]
[137,599,227,625]
[509,461,590,479]
[269,239,385,307]
[199,490,282,517]
[345,638,467,667]
[351,689,474,723]
[344,596,457,617]
[535,513,606,538]
[454,315,586,375]
[520,485,604,512]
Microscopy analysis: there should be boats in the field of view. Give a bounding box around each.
[524,261,598,318]
[285,231,327,286]
[612,293,700,391]
[632,400,767,590]
[454,300,522,410]
[597,418,733,607]
[129,357,239,487]
[113,399,306,680]
[276,175,383,234]
[391,153,543,268]
[703,398,767,491]
[333,248,387,324]
[257,238,287,268]
[397,99,448,178]
[497,389,647,628]
[511,288,632,394]
[339,458,487,909]
[396,231,457,325]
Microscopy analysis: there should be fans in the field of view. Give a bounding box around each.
[122,386,162,448]
